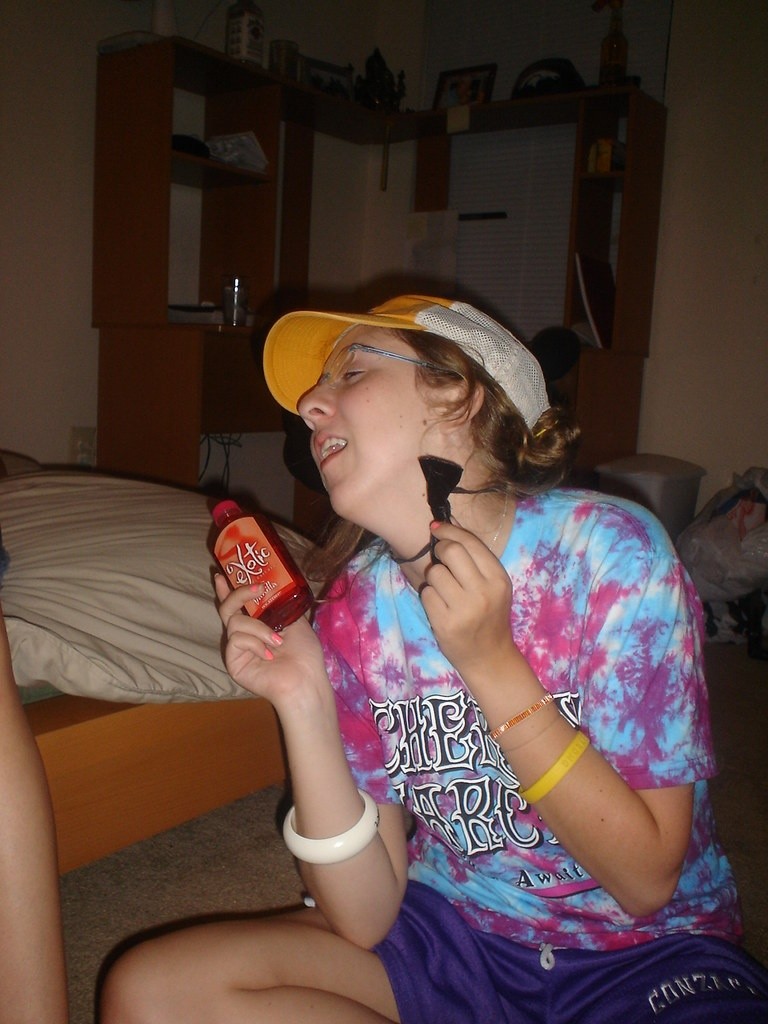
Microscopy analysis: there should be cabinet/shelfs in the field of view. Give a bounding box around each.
[89,44,670,543]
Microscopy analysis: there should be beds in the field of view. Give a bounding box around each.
[0,449,321,874]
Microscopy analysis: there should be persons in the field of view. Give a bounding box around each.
[0,544,69,1024]
[98,296,768,1024]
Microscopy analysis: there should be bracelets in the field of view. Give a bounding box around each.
[518,731,591,804]
[283,788,379,864]
[490,692,554,740]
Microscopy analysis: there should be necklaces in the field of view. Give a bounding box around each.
[489,495,507,550]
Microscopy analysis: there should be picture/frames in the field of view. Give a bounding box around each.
[432,63,497,109]
[302,55,355,98]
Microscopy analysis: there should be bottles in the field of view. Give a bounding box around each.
[151,0,175,37]
[226,0,264,65]
[598,7,628,82]
[211,499,313,632]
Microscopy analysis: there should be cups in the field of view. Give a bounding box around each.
[268,39,300,82]
[221,274,250,327]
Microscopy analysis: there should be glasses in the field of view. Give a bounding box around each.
[318,341,456,390]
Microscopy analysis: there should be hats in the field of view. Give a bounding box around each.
[262,295,550,437]
